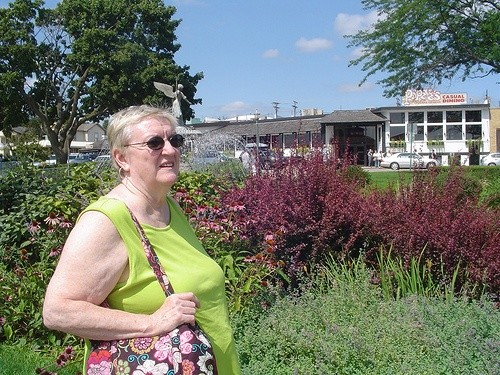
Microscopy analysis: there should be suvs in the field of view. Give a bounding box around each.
[239,142,287,169]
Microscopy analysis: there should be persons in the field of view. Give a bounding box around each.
[367,148,391,168]
[429,149,437,159]
[42,105,241,375]
[413,149,422,168]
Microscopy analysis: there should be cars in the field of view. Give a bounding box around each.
[380,152,439,170]
[0,152,112,169]
[481,153,500,166]
[197,150,222,164]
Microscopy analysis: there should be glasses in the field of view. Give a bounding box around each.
[124,134,185,150]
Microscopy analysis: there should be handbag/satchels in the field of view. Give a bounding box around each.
[86,198,218,375]
